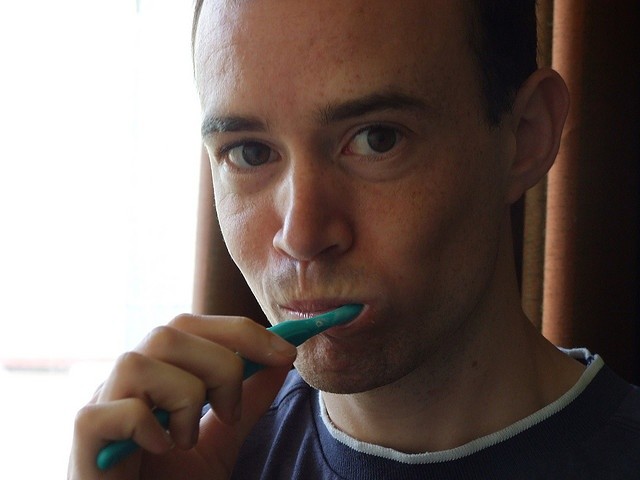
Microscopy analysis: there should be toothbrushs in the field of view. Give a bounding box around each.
[96,300,362,473]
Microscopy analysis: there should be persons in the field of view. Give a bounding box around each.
[66,0,639,480]
